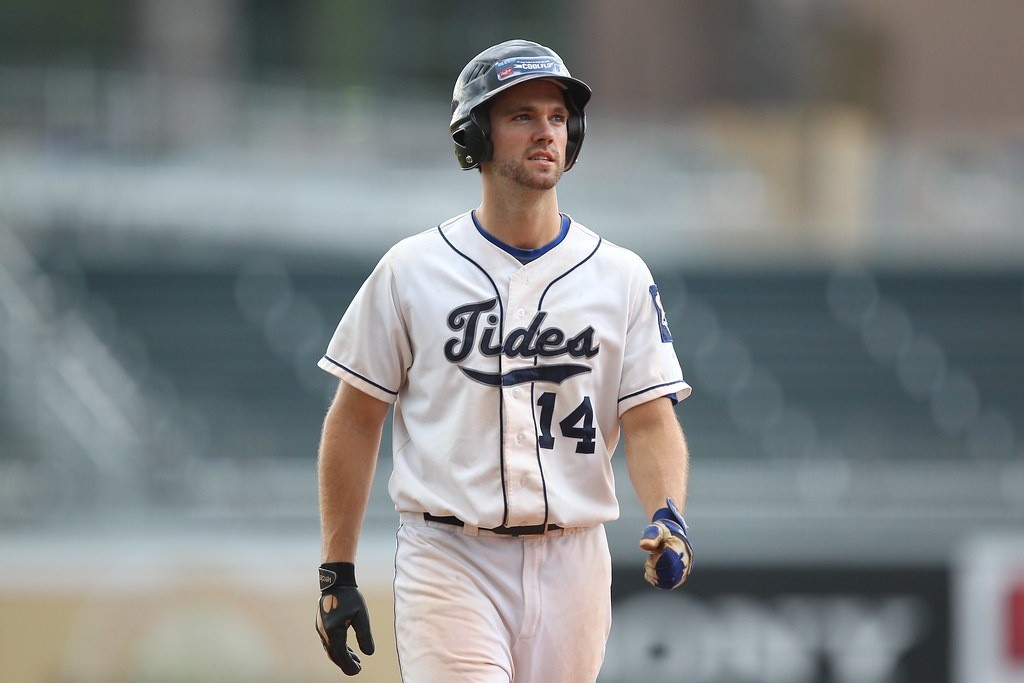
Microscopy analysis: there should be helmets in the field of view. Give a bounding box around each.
[447,39,593,173]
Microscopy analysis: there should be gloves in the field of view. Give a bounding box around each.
[316,561,376,676]
[639,497,694,591]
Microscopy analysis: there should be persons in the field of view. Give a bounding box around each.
[314,38,694,682]
